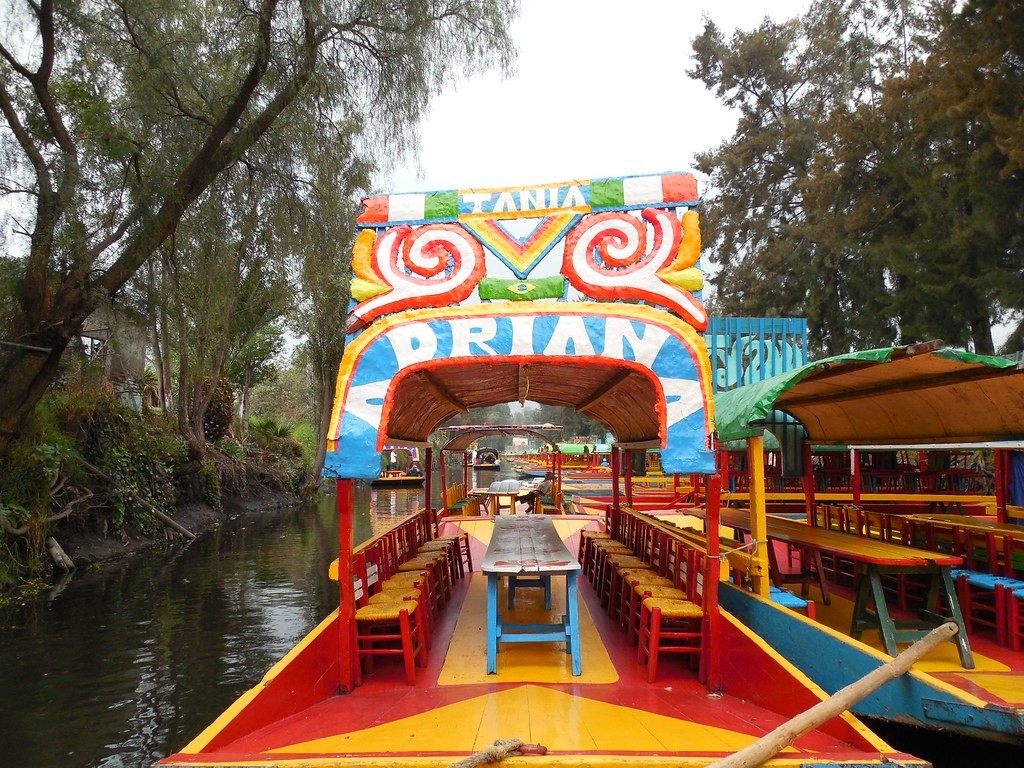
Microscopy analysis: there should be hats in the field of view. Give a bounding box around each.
[603,458,606,461]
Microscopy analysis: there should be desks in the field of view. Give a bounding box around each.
[681,507,976,669]
[480,513,582,676]
[913,515,1024,555]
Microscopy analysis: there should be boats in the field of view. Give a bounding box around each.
[510,458,619,495]
[473,447,500,470]
[372,448,425,486]
[652,338,1023,748]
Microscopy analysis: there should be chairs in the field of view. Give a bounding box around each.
[328,483,1024,695]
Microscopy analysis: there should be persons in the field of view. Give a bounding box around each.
[481,454,502,464]
[406,462,423,477]
[467,450,477,463]
[515,472,552,513]
[601,459,609,467]
[390,449,397,470]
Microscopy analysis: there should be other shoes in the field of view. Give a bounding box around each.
[515,495,518,502]
